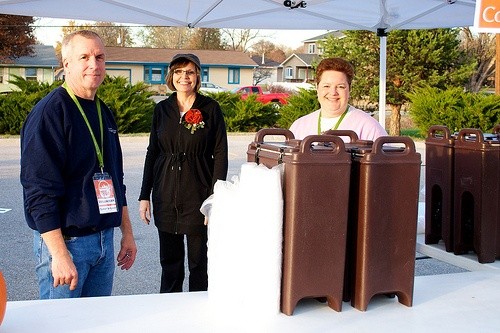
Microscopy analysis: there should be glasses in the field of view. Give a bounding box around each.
[173,70,196,74]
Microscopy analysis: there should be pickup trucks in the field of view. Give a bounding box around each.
[231,85,292,107]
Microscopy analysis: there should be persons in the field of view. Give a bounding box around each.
[20,30,138,300]
[289,58,391,146]
[138,53,228,293]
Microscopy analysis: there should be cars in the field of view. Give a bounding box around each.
[199,82,227,93]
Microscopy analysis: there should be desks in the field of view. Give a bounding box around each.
[0,202,500,333]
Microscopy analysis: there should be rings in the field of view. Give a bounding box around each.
[126,254,129,257]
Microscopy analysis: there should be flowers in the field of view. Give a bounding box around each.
[178,108,205,134]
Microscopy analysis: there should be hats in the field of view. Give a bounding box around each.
[168,53,201,70]
